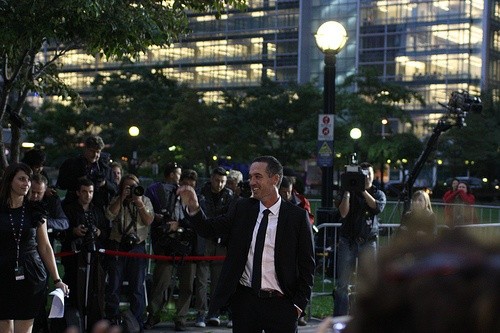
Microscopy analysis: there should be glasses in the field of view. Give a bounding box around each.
[16,175,34,183]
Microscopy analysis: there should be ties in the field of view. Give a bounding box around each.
[252,208,270,289]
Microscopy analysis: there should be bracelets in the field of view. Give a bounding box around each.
[137,203,145,210]
[53,279,62,285]
[344,193,350,197]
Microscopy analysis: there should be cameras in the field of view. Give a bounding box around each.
[83,225,97,252]
[127,185,144,198]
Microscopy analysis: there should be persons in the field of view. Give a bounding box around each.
[175,155,315,333]
[0,135,315,333]
[398,189,436,238]
[332,162,387,315]
[442,180,477,228]
[313,228,500,333]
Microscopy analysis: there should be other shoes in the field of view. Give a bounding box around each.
[298,316,308,326]
[209,314,221,326]
[144,316,162,328]
[195,314,206,327]
[174,316,187,330]
